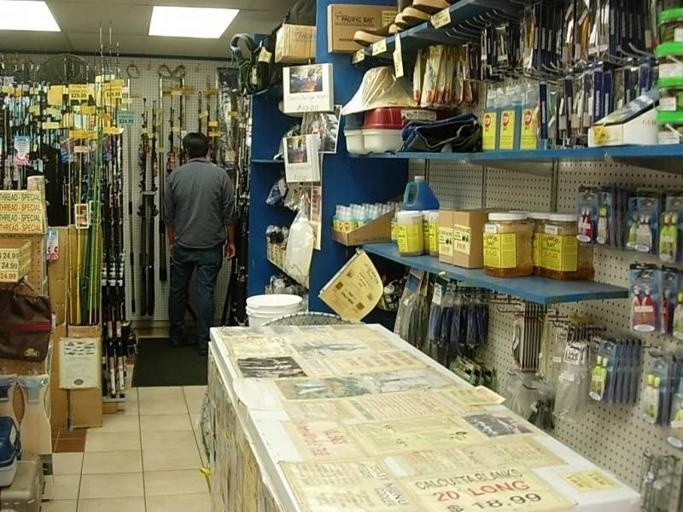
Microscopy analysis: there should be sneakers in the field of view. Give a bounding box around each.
[170,337,209,356]
[387,1,451,36]
[351,21,393,46]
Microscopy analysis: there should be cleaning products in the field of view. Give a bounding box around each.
[481,84,543,151]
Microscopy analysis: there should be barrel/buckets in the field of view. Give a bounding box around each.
[245,293,303,327]
[401,175,439,210]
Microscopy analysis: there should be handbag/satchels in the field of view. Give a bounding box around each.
[0,278,51,363]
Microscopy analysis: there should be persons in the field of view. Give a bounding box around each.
[164,133,239,357]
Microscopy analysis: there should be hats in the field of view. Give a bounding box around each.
[338,68,420,117]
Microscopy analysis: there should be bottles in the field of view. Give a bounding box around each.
[482,206,594,282]
[333,201,399,233]
[651,8,682,146]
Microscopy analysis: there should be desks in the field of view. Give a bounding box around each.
[203,324,643,512]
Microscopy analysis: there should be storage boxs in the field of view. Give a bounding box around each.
[2,223,102,444]
